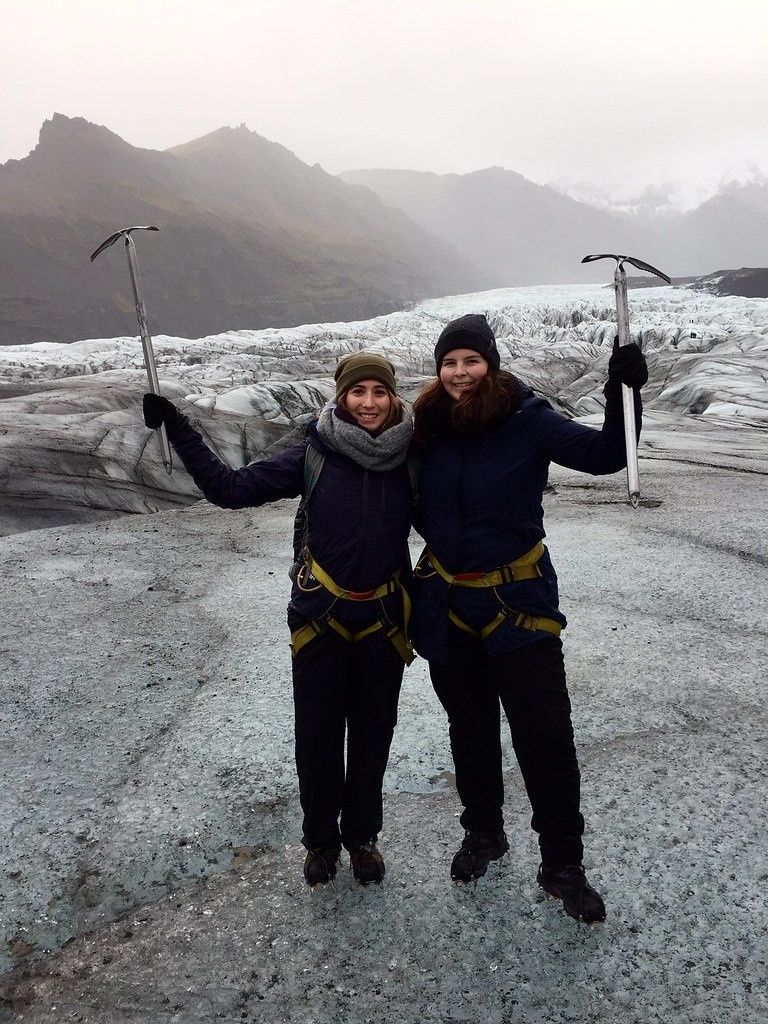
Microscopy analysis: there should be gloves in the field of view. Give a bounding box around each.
[142,392,190,440]
[600,335,649,401]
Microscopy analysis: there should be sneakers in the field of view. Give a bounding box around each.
[535,859,607,924]
[302,847,343,887]
[352,841,386,885]
[451,831,510,883]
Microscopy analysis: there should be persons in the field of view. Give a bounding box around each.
[411,314,649,924]
[142,352,419,888]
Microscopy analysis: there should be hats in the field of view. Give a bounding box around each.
[333,351,397,400]
[434,313,499,378]
